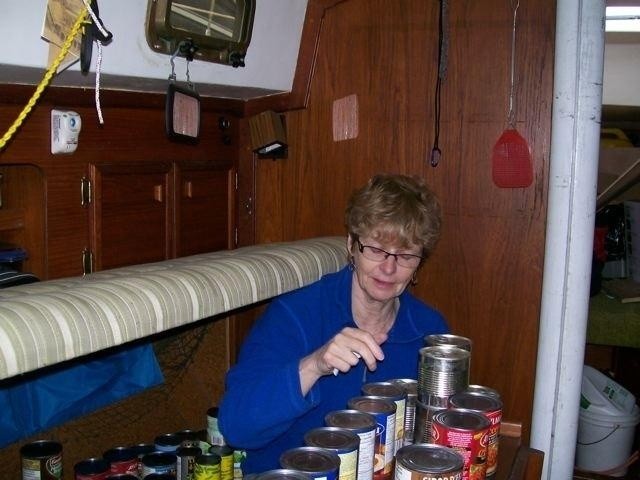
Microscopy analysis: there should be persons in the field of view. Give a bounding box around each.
[217,173,452,477]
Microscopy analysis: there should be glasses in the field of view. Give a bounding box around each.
[356,238,424,268]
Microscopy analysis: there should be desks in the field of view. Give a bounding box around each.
[484,441,545,480]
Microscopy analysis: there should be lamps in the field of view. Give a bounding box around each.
[248,111,286,157]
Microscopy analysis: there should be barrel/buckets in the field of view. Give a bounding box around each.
[577,408,639,476]
[580,365,636,415]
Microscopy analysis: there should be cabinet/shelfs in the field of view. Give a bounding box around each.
[0,162,49,289]
[79,160,239,275]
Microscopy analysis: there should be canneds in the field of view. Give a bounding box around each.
[20,407,246,480]
[249,333,502,480]
